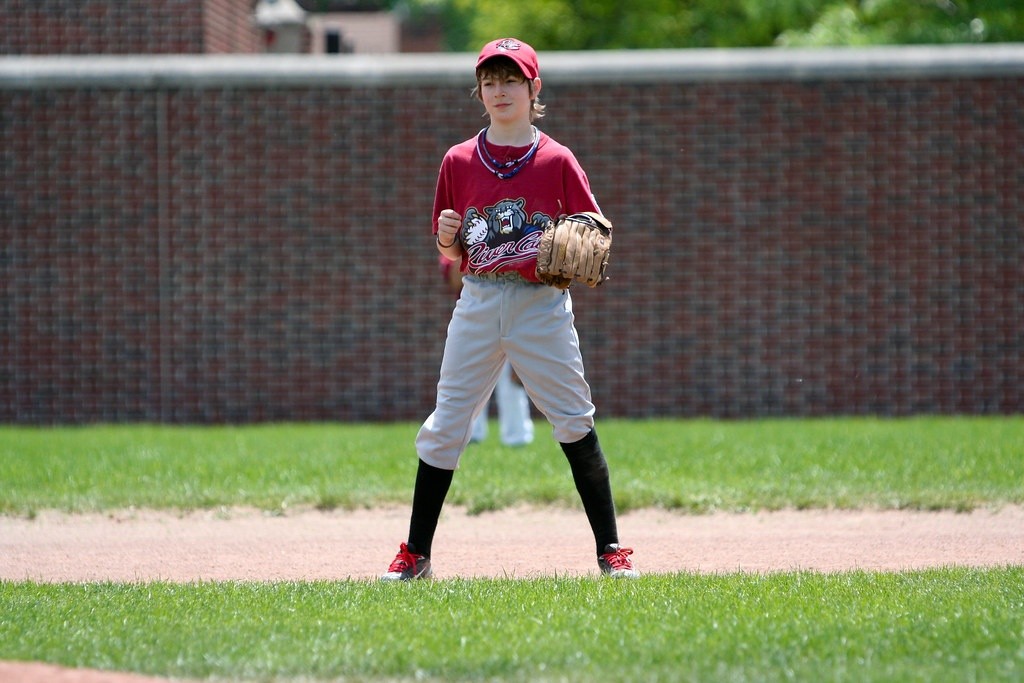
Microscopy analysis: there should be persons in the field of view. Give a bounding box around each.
[437,256,535,448]
[386,38,640,581]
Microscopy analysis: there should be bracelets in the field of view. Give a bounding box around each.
[437,233,457,248]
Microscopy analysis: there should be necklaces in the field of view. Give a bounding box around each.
[476,126,540,178]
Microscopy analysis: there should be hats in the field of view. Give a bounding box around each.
[476,38,539,80]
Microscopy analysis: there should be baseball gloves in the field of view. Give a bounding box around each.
[535,211,614,289]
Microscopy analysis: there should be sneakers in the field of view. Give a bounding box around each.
[597,543,637,579]
[382,542,431,582]
